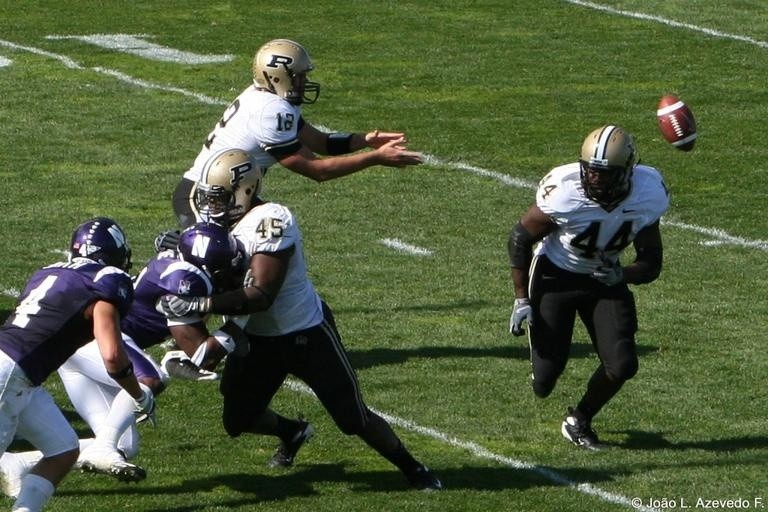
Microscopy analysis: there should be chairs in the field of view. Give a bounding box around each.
[160,356,220,381]
[70,437,147,484]
[268,419,313,470]
[560,406,609,452]
[408,464,443,493]
[1,452,22,499]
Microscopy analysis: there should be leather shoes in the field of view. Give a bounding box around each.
[161,292,214,319]
[509,297,535,337]
[590,256,628,289]
[154,230,181,253]
[131,389,159,430]
[243,269,254,288]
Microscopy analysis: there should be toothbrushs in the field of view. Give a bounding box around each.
[657,95,695,151]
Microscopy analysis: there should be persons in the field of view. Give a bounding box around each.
[156,147,443,492]
[508,125,671,453]
[0,222,255,498]
[160,39,424,382]
[0,217,158,511]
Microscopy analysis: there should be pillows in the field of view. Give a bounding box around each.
[193,148,262,226]
[578,123,635,206]
[175,221,242,286]
[252,39,320,106]
[68,218,133,273]
